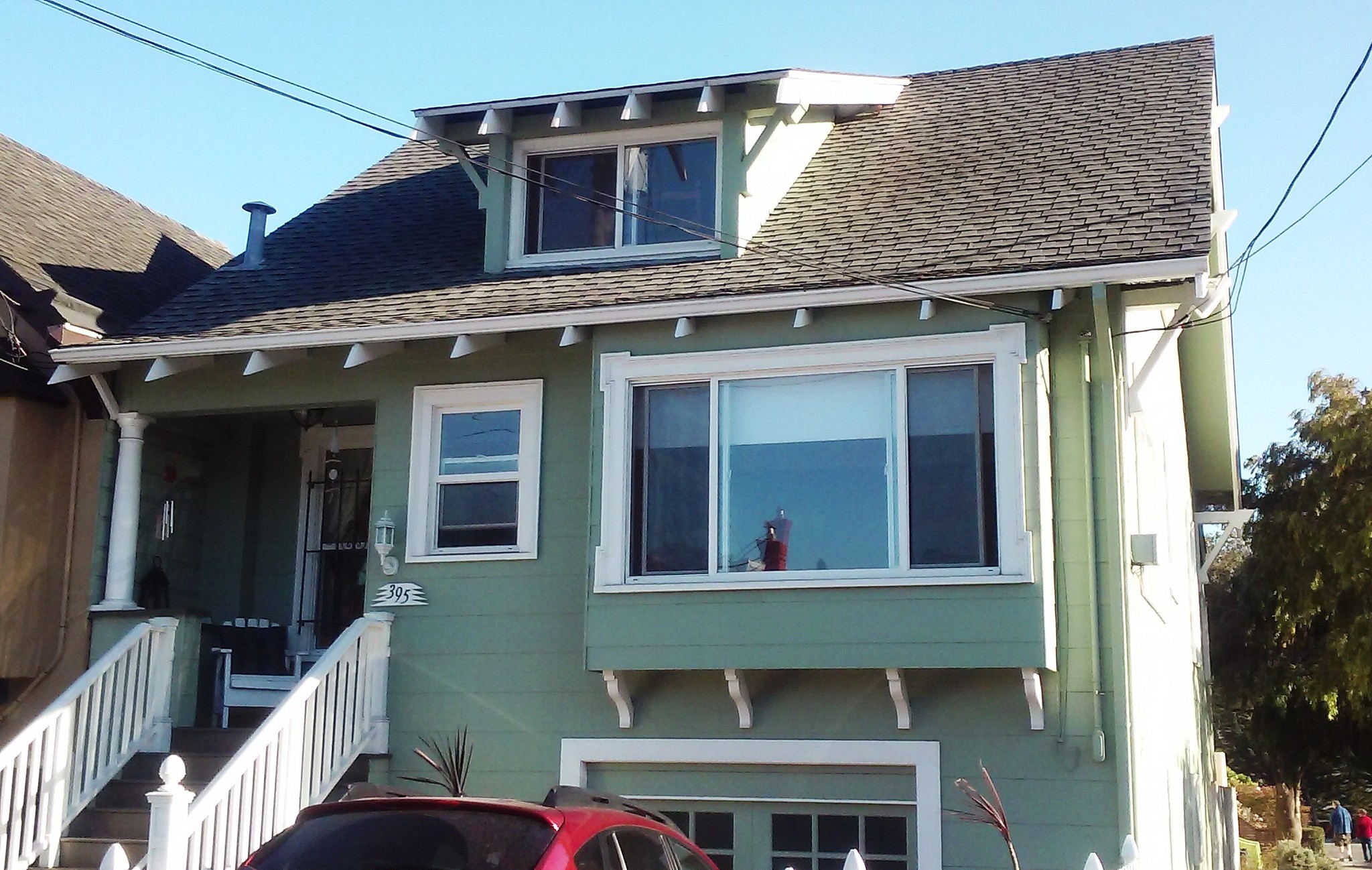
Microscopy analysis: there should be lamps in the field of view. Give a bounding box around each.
[1210,750,1229,790]
[375,510,399,577]
[289,408,327,432]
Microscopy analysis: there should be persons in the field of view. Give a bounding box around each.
[1330,800,1352,862]
[1354,808,1372,863]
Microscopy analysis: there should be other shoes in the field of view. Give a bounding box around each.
[1365,859,1371,863]
[1349,856,1353,861]
[1340,858,1344,861]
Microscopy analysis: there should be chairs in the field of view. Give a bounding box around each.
[212,618,309,731]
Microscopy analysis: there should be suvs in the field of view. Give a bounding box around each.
[236,780,720,870]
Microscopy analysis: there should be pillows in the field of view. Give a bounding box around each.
[201,624,288,676]
